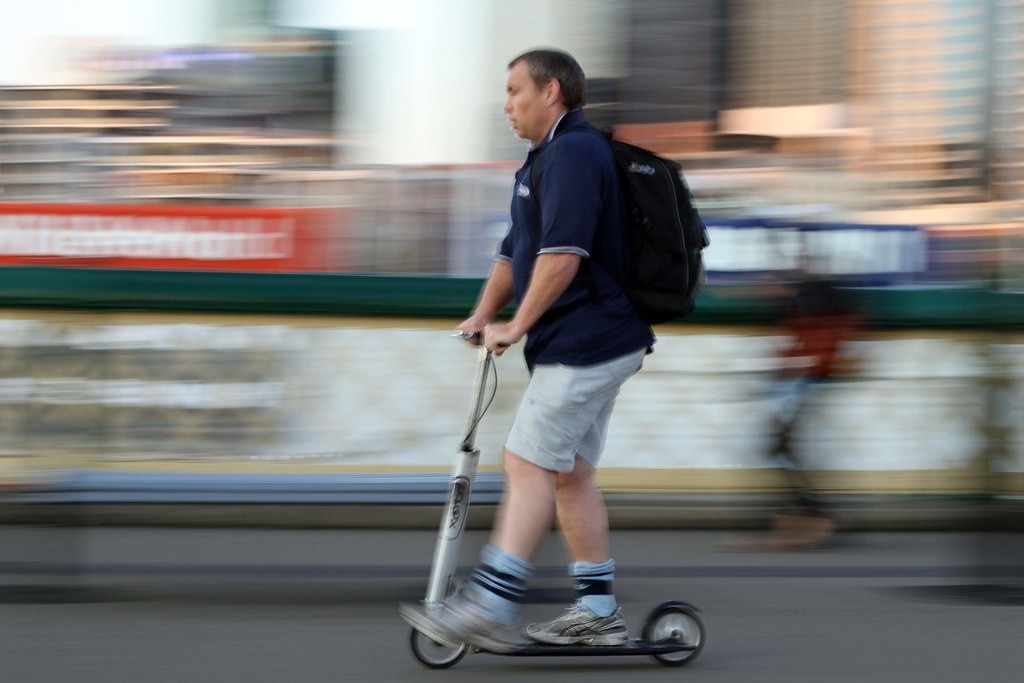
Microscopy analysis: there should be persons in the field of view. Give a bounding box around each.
[398,50,656,656]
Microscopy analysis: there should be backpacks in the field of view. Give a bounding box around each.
[530,124,710,321]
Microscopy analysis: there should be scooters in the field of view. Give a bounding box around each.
[407,330,710,670]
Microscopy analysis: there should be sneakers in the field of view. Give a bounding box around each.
[398,580,527,654]
[525,600,630,648]
